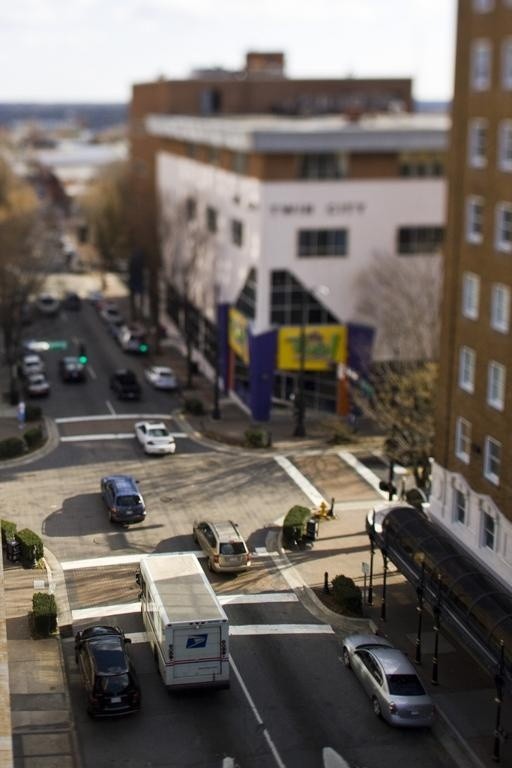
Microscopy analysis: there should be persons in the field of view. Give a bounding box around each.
[326,497,338,517]
[316,499,329,517]
